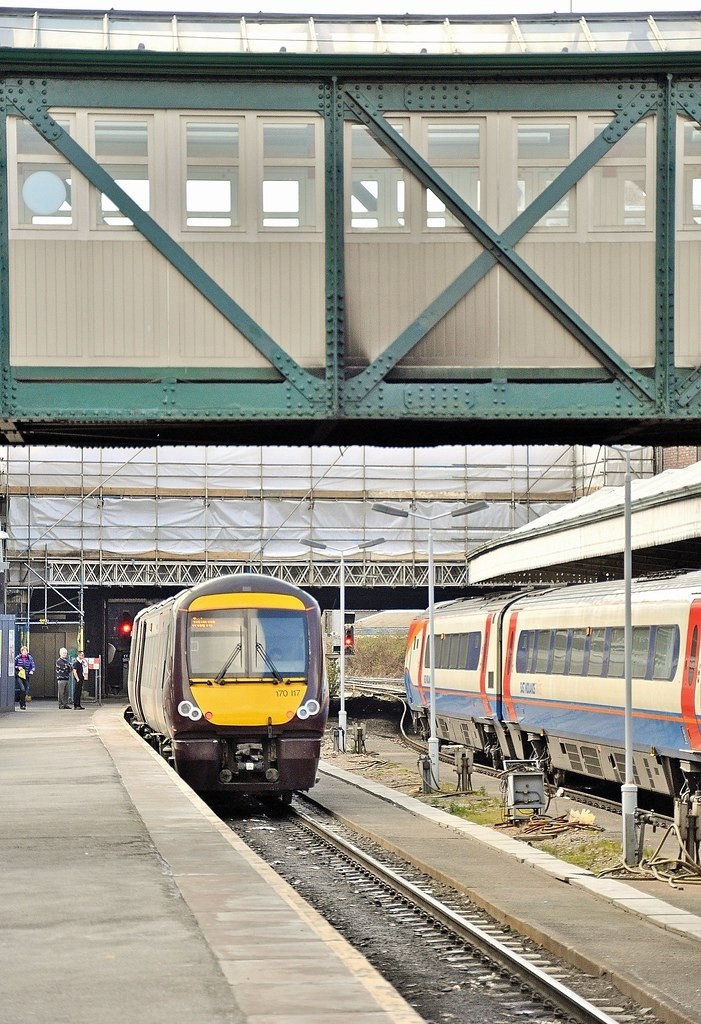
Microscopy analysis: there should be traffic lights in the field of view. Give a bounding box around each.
[343,625,354,650]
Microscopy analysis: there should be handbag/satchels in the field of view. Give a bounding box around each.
[17,668,26,679]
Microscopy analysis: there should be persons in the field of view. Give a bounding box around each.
[55,648,73,709]
[72,651,85,710]
[14,646,35,710]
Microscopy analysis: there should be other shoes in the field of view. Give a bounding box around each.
[20,706,26,710]
[74,706,85,710]
[59,705,72,709]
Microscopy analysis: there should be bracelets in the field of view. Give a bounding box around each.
[31,670,34,673]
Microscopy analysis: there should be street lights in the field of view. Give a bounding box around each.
[370,500,488,791]
[299,537,386,752]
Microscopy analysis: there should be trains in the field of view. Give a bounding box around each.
[127,572,329,806]
[403,568,701,800]
